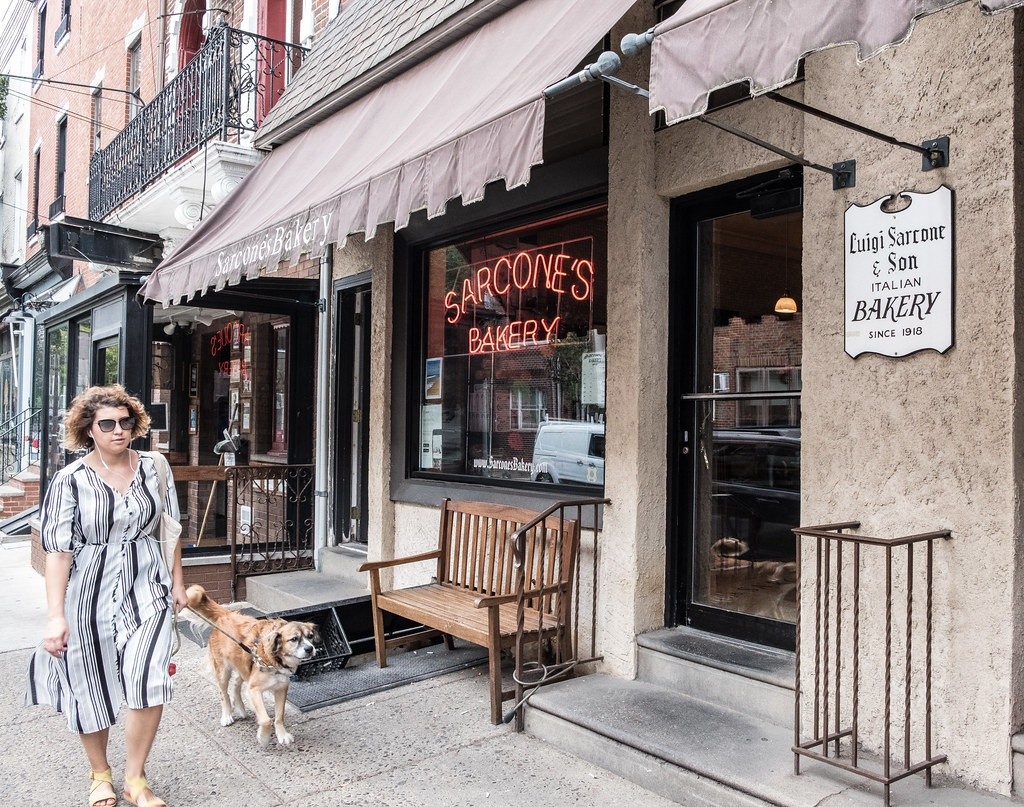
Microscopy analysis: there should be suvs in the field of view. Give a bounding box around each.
[710,424,801,562]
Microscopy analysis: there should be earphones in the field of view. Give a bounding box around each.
[89,430,94,439]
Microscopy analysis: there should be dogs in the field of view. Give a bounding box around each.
[183,584,320,747]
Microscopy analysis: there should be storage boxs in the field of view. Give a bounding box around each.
[270,606,353,681]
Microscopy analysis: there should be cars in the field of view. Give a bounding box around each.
[30,422,65,463]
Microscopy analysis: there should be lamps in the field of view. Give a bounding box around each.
[163,306,245,336]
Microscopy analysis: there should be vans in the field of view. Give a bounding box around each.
[530,412,606,487]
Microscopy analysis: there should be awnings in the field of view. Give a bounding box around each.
[136,0,637,310]
[620,0,1024,126]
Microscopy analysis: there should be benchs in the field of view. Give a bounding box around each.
[356,495,580,724]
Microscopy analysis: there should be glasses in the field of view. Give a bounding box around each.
[92,416,137,433]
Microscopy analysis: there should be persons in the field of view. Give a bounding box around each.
[24,383,189,807]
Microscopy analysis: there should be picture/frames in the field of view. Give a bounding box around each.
[187,404,198,435]
[188,362,199,398]
[228,319,254,433]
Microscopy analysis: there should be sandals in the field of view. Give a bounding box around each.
[122,772,168,807]
[88,765,118,807]
